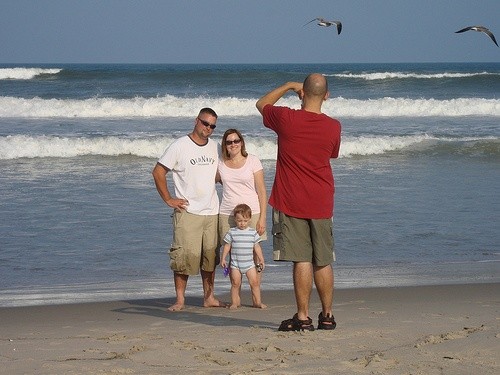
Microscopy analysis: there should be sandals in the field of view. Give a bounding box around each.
[318,312,336,330]
[278,313,315,331]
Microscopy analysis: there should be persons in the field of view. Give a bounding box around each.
[255,73,341,332]
[220,203,266,310]
[152,107,226,311]
[216,128,268,307]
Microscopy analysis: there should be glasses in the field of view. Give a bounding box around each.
[225,140,241,145]
[198,118,216,129]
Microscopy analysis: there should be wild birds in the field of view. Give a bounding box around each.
[304,17,343,35]
[454,25,500,48]
